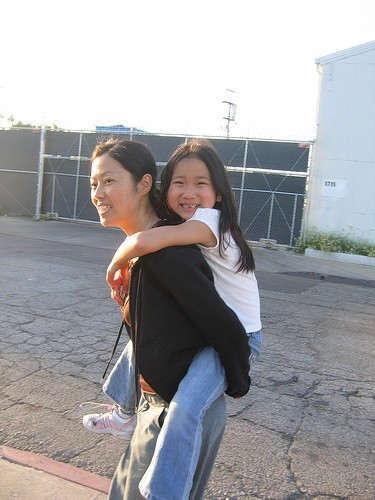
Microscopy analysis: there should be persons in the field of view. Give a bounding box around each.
[81,138,263,500]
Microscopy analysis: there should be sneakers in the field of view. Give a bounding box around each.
[83,404,137,440]
